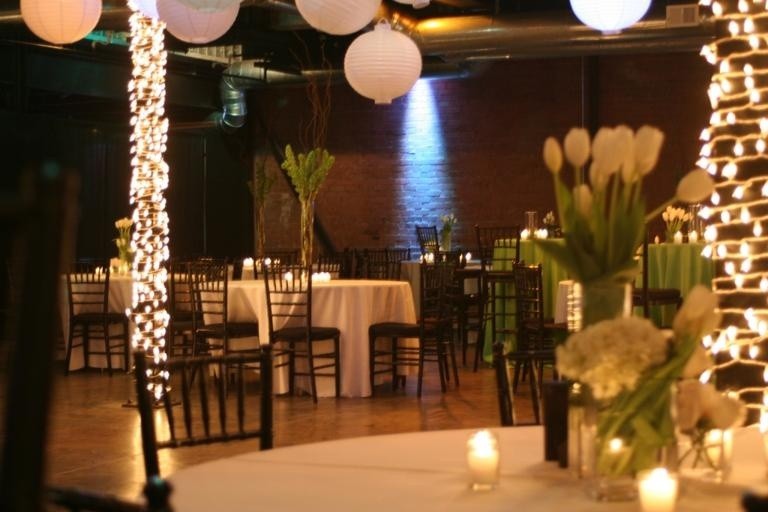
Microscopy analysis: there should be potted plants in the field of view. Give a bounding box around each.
[280,144,335,275]
[245,160,275,258]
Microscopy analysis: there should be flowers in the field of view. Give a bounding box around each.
[530,126,715,327]
[116,217,133,257]
[543,212,555,227]
[441,214,457,248]
[552,287,741,472]
[662,206,688,241]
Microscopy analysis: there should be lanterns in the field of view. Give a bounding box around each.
[289,1,384,37]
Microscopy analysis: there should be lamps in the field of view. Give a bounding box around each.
[294,0,380,35]
[394,0,430,9]
[421,53,469,78]
[255,62,305,76]
